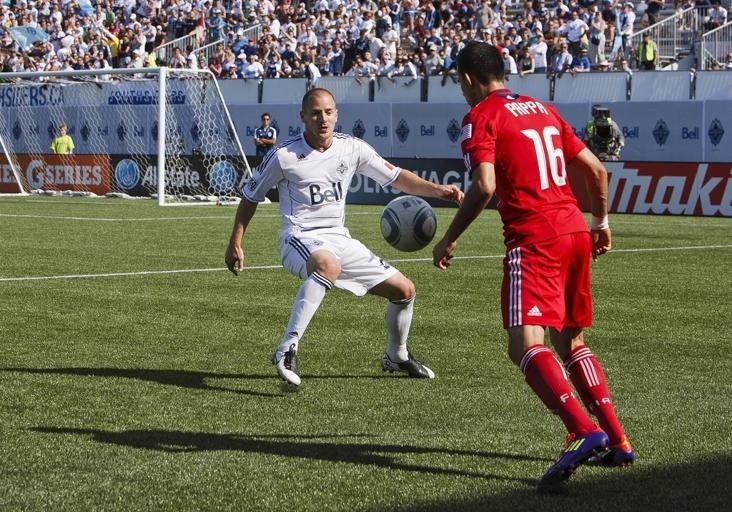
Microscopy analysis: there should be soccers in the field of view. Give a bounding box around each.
[380,196,437,252]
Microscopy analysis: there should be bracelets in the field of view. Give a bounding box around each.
[590,213,609,231]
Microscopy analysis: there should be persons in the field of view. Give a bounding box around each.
[253,112,276,156]
[434,43,634,482]
[0,0,732,83]
[50,123,74,154]
[583,103,626,160]
[224,88,466,386]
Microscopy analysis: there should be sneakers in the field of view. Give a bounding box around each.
[382,349,435,379]
[541,431,635,482]
[270,350,301,386]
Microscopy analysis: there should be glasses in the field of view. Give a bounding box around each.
[0,0,613,68]
[263,119,269,120]
